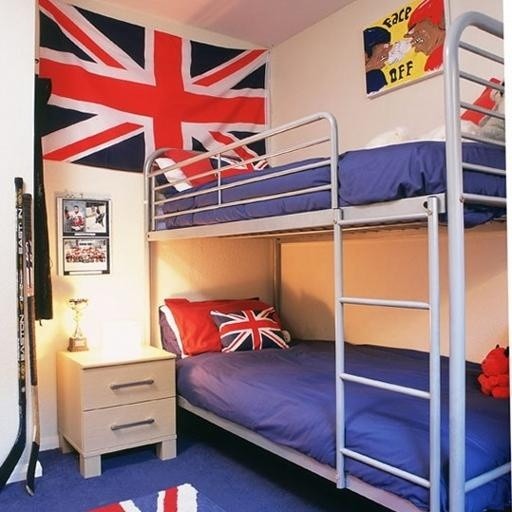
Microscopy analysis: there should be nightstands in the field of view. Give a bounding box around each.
[56,343,177,478]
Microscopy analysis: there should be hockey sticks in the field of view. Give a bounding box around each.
[0,177,27,489]
[25,192,40,495]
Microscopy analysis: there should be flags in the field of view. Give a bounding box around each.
[37,0,271,173]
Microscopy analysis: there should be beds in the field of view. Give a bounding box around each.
[142,9,512,512]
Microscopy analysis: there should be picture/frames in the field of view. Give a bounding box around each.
[56,196,110,275]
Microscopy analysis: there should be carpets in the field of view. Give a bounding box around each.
[85,481,230,512]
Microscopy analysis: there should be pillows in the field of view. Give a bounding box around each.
[151,146,271,195]
[159,296,296,359]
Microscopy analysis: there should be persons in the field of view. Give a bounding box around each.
[65,205,84,226]
[66,246,106,263]
[95,209,105,227]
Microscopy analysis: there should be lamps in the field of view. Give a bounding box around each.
[64,295,91,352]
